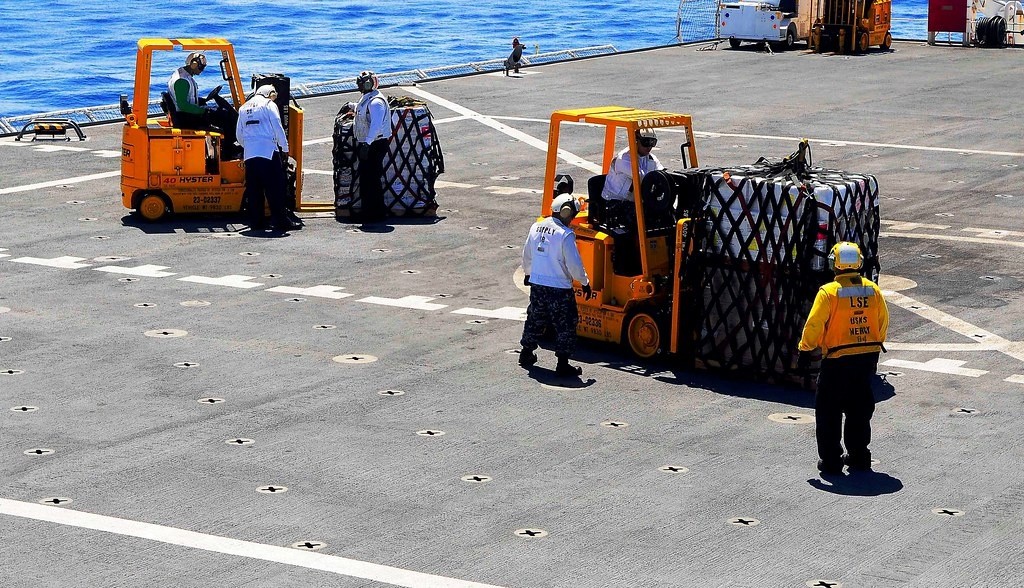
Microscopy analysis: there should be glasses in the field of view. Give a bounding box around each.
[198,64,205,71]
[639,137,657,147]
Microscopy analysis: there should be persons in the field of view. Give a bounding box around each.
[337,71,393,230]
[167,53,240,161]
[519,193,593,379]
[600,127,664,262]
[796,240,889,473]
[236,85,304,229]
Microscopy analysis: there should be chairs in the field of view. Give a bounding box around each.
[587,174,629,240]
[160,91,207,128]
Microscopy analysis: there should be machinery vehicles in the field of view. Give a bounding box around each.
[799,0,892,55]
[540,106,702,363]
[118,38,335,221]
[719,0,824,48]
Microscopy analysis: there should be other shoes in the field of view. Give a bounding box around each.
[275,221,302,230]
[840,454,871,468]
[251,222,274,230]
[556,363,582,376]
[518,349,537,366]
[360,223,387,232]
[817,459,842,474]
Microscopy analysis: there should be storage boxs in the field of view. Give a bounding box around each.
[698,162,882,381]
[332,98,435,220]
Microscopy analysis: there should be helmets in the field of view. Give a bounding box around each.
[185,52,207,71]
[828,241,864,275]
[551,193,581,223]
[356,71,378,94]
[635,128,658,144]
[256,84,278,101]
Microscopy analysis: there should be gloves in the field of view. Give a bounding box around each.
[524,275,530,286]
[796,351,812,370]
[582,283,591,301]
